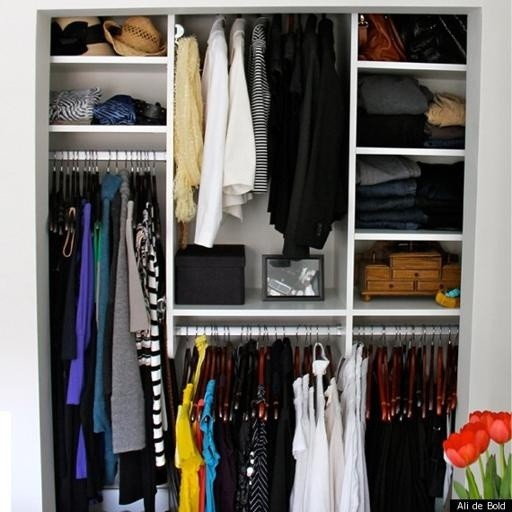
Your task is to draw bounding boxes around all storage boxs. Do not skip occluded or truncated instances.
[174,244,245,307]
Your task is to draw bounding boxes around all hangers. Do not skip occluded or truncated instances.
[174,323,461,422]
[48,149,162,235]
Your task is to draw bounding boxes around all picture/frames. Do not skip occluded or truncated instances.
[261,255,324,302]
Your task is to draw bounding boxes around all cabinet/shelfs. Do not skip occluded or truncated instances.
[37,8,482,511]
[361,253,460,300]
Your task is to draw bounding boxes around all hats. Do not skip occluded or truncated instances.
[104,16,167,56]
[50,16,114,54]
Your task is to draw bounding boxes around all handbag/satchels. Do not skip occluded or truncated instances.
[49,87,102,125]
[358,14,466,64]
[94,95,138,125]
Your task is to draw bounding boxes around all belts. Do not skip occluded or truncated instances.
[139,102,166,125]
